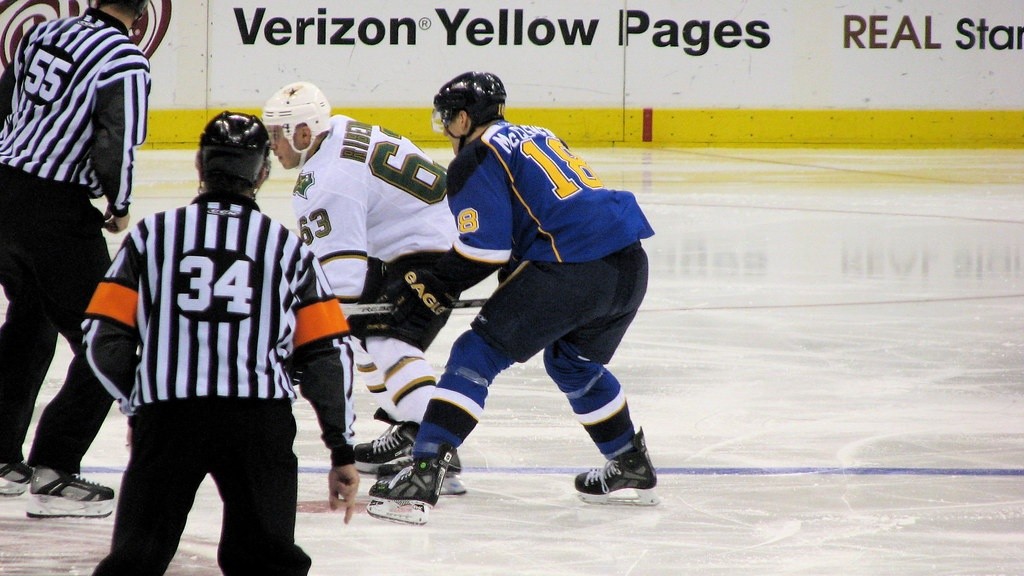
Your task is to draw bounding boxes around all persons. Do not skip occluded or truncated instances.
[81,110,359,576]
[370,72,657,510]
[0,0,151,502]
[261,82,461,479]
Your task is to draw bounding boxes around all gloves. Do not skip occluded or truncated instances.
[381,270,460,338]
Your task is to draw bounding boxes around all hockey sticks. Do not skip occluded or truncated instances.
[339,297,488,319]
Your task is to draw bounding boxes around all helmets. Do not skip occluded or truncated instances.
[199,111,271,184]
[262,82,331,136]
[121,1,149,16]
[434,72,508,124]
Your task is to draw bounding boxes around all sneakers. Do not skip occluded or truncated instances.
[352,421,422,474]
[574,429,656,506]
[377,449,466,495]
[367,441,458,524]
[0,461,35,494]
[27,464,114,518]
